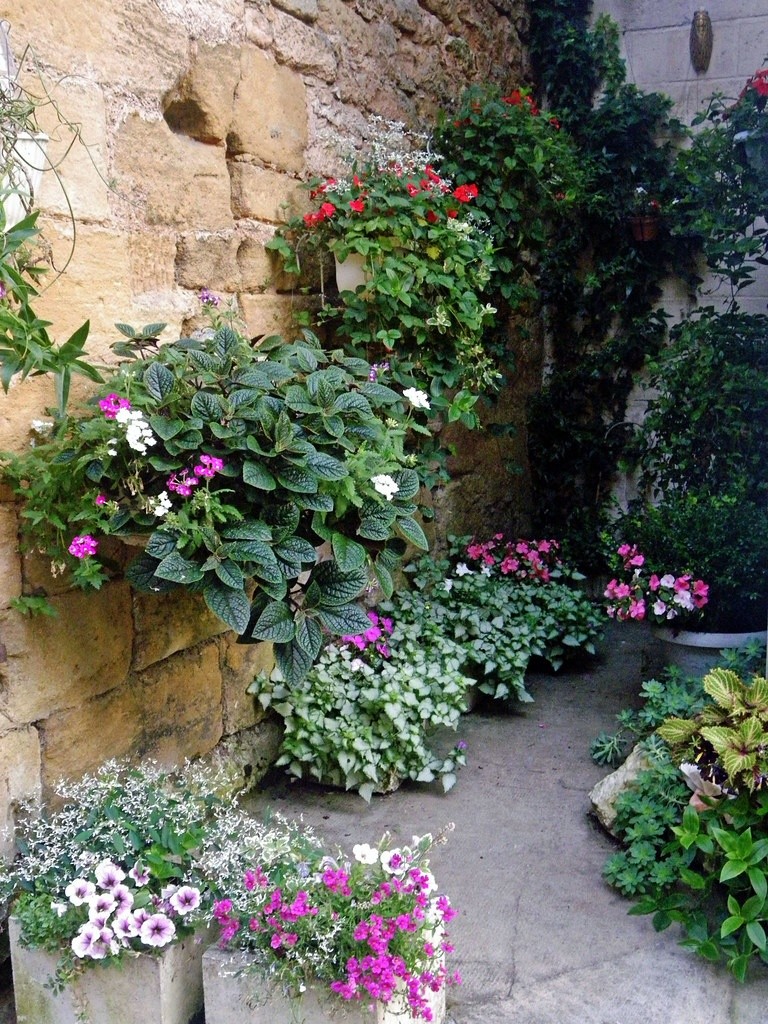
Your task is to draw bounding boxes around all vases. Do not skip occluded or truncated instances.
[330,252,374,301]
[648,629,768,688]
[631,219,660,241]
[0,132,54,234]
[735,138,768,170]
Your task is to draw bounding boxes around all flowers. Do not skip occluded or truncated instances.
[0,0,768,1024]
